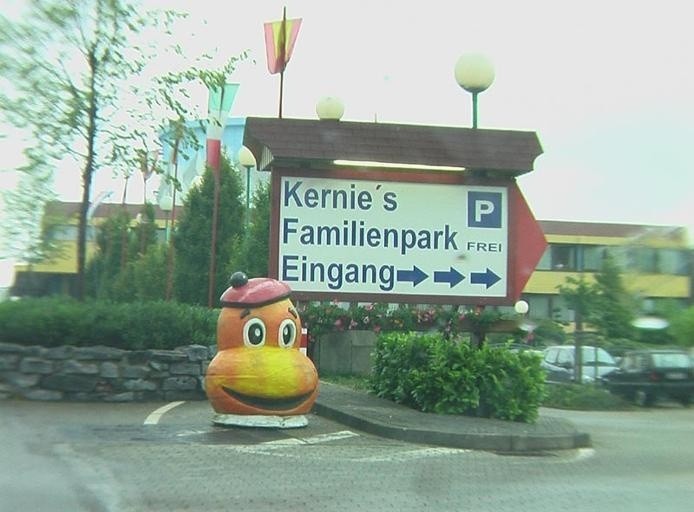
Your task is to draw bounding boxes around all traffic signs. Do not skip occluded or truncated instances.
[267,166,548,306]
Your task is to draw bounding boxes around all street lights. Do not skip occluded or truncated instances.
[238,146,257,230]
[515,300,529,320]
[159,195,173,244]
[455,51,495,129]
[316,95,345,121]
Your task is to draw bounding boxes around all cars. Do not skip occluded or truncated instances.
[509,344,694,407]
